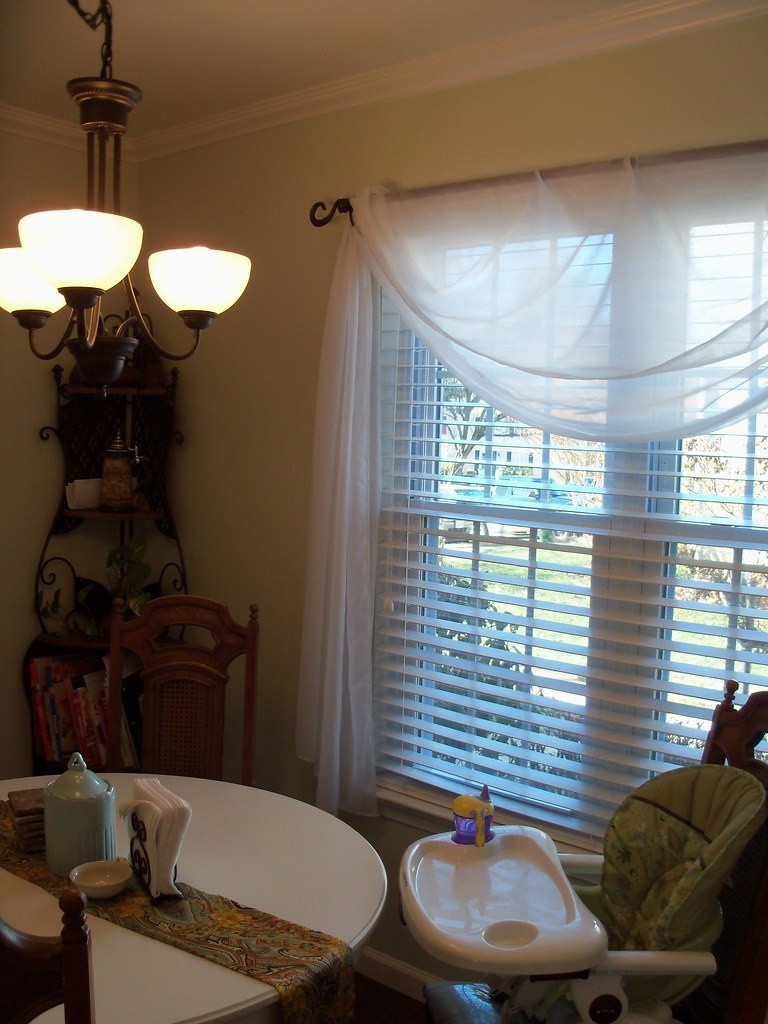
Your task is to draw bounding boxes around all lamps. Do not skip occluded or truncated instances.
[0,0,251,362]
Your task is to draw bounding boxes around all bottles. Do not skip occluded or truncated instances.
[453,784,494,847]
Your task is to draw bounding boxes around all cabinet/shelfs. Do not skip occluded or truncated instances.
[21,308,188,774]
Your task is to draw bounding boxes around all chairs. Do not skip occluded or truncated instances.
[0,885,94,1024]
[421,679,768,1024]
[479,763,768,1024]
[104,589,263,787]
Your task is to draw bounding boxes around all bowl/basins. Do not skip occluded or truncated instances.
[69,860,133,898]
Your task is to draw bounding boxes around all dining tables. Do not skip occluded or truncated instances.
[0,773,388,1023]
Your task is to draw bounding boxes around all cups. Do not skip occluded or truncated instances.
[43,753,117,876]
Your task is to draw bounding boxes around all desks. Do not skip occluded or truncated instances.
[399,818,609,982]
